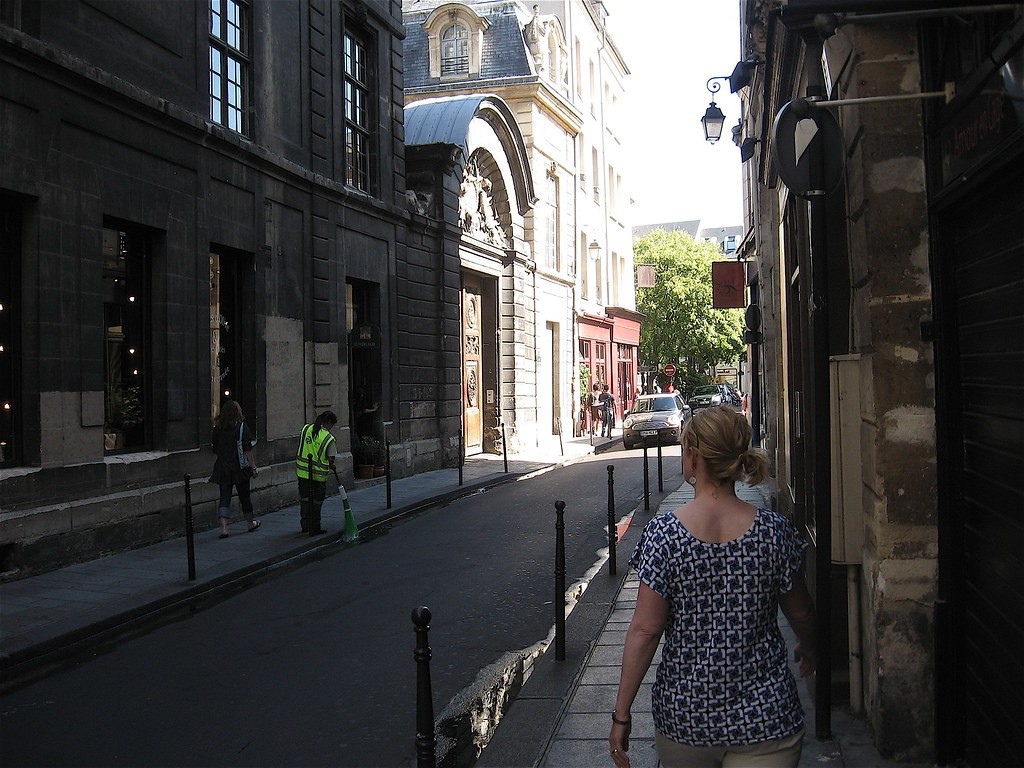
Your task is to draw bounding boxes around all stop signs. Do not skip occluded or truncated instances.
[664,364,676,377]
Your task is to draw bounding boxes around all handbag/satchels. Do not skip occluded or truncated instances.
[237,422,251,467]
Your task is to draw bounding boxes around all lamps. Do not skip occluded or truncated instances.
[701,60,765,164]
[588,238,601,263]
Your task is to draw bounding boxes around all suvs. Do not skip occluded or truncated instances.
[688,385,723,414]
[623,390,692,447]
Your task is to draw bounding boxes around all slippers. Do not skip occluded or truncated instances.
[247,519,261,532]
[219,533,230,539]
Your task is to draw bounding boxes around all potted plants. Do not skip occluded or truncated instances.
[352,436,388,479]
[105,386,143,450]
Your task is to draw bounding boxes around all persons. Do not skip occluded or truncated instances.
[591,384,617,438]
[653,386,658,401]
[209,399,263,539]
[296,410,337,536]
[606,405,820,768]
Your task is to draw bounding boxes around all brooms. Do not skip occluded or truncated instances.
[332,469,360,543]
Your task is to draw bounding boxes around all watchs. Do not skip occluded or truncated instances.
[611,710,632,725]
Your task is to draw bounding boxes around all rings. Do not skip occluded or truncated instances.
[615,749,618,752]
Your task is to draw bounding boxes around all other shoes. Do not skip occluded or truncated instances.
[302,528,327,536]
[592,429,613,438]
